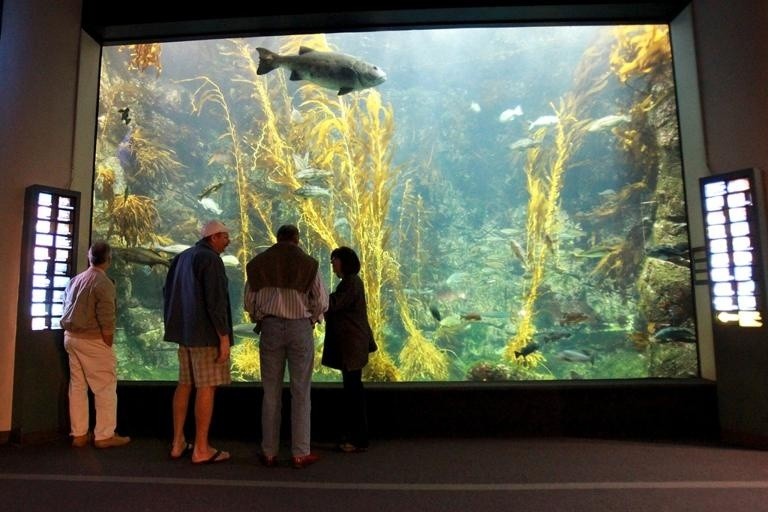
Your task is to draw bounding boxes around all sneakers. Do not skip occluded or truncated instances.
[338,442,369,454]
[71,433,88,449]
[94,433,131,450]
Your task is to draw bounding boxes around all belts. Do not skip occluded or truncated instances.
[262,315,279,320]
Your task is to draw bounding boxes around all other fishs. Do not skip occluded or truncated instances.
[465,223,694,365]
[468,99,482,112]
[496,102,632,151]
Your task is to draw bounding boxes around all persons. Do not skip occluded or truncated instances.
[244,225,330,469]
[60,243,131,448]
[163,219,234,463]
[322,247,378,452]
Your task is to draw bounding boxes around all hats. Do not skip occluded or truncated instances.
[200,218,232,239]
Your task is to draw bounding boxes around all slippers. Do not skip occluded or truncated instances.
[170,439,194,462]
[191,449,232,466]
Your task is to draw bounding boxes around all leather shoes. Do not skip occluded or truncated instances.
[290,453,322,469]
[262,455,278,467]
